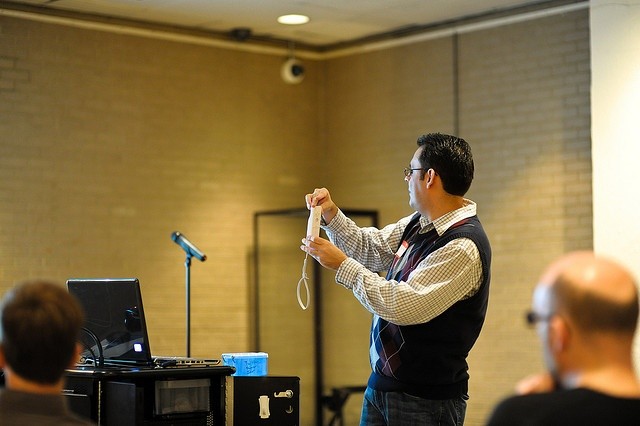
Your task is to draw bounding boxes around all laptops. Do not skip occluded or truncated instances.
[65,280,220,366]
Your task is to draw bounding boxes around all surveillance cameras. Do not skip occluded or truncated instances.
[281,58,312,84]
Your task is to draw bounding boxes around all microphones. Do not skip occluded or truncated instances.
[171,230,208,260]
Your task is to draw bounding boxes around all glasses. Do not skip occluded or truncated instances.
[404,167,438,177]
[527,312,572,331]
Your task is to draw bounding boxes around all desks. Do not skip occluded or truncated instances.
[63,366,299,426]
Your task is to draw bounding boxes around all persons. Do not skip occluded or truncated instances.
[300,132,492,425]
[0,280,96,426]
[484,250,640,426]
